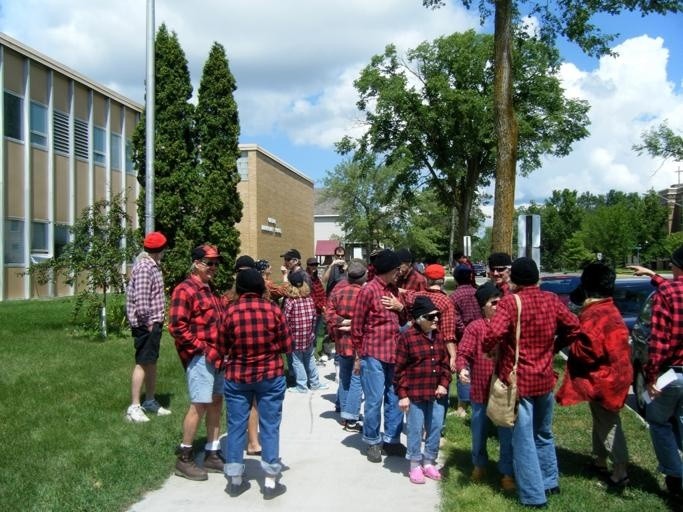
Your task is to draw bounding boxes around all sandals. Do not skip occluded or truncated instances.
[606,478,630,492]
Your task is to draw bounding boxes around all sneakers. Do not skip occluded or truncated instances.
[289,387,306,392]
[143,401,172,415]
[471,465,485,482]
[344,422,441,483]
[321,384,329,389]
[501,476,515,492]
[127,405,150,422]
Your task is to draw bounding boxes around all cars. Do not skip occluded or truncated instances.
[472,264,486,277]
[562,275,678,326]
[535,271,583,306]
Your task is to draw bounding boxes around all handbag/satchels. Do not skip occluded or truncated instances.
[486,379,518,428]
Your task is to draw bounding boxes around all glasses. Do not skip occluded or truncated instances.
[201,261,219,266]
[423,312,440,320]
[337,254,344,257]
[284,258,296,261]
[490,267,507,272]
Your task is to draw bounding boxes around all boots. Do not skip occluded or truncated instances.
[175,446,286,499]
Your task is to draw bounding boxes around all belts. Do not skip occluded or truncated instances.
[662,367,682,372]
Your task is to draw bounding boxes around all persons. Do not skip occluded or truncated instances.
[216,269,295,500]
[126,231,172,422]
[450,251,582,507]
[325,247,456,483]
[627,245,683,495]
[169,245,227,481]
[256,249,329,393]
[220,256,269,455]
[555,260,634,487]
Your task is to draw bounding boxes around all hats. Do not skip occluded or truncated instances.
[236,256,269,294]
[192,245,220,260]
[425,265,446,279]
[144,232,167,252]
[412,296,437,318]
[280,249,318,287]
[671,247,683,268]
[454,252,538,306]
[347,249,411,283]
